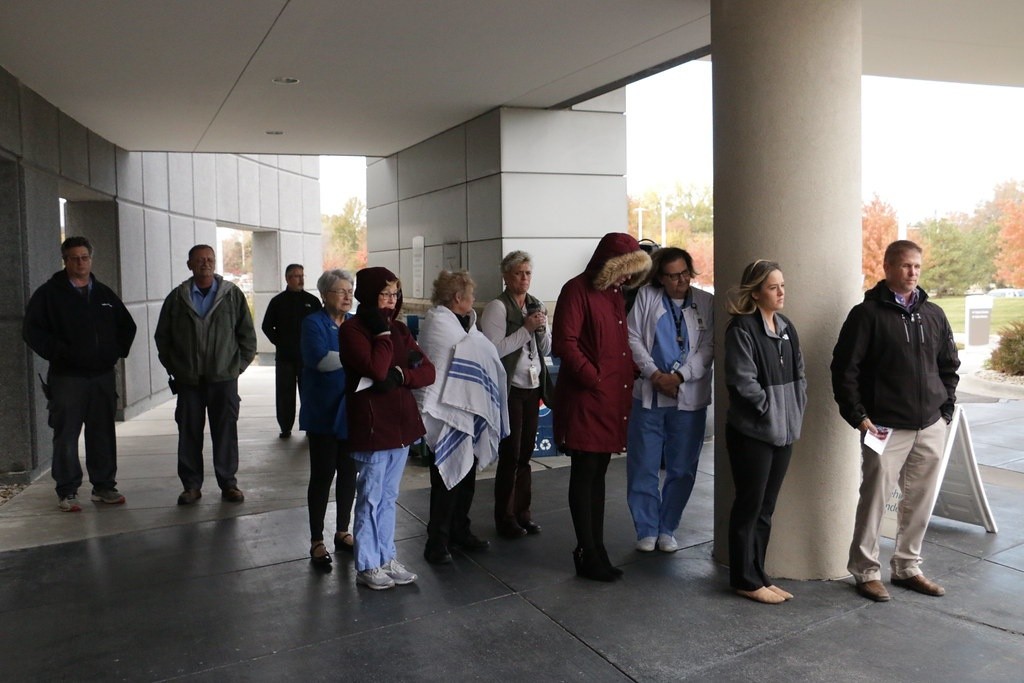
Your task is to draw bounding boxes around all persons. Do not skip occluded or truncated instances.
[626,247,713,552]
[830,240,961,602]
[480,249,554,538]
[155,245,257,504]
[23,236,137,511]
[339,266,436,591]
[724,259,807,603]
[262,264,322,438]
[413,269,510,567]
[300,269,356,566]
[551,233,654,580]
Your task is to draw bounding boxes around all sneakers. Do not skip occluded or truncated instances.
[381,559,417,584]
[91,487,125,502]
[58,493,82,512]
[356,567,395,590]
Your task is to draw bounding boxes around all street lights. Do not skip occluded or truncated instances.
[635,208,645,244]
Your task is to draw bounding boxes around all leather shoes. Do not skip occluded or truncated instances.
[424,533,490,562]
[891,575,945,596]
[334,532,355,547]
[495,521,541,536]
[310,543,332,564]
[856,580,891,600]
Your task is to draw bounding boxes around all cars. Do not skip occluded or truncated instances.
[236,275,253,292]
[988,288,1024,298]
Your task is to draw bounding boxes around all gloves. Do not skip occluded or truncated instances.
[366,307,390,334]
[372,367,403,392]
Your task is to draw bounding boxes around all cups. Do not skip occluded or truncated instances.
[528,302,543,332]
[407,350,424,368]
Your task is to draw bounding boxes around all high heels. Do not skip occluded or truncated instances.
[597,544,623,576]
[574,545,615,581]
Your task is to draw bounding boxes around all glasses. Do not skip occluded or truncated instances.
[661,268,690,280]
[65,255,91,261]
[380,289,400,299]
[326,288,353,296]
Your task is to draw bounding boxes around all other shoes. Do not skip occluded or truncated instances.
[222,487,244,498]
[659,533,678,552]
[178,488,202,503]
[737,585,793,602]
[279,429,291,438]
[636,536,657,551]
